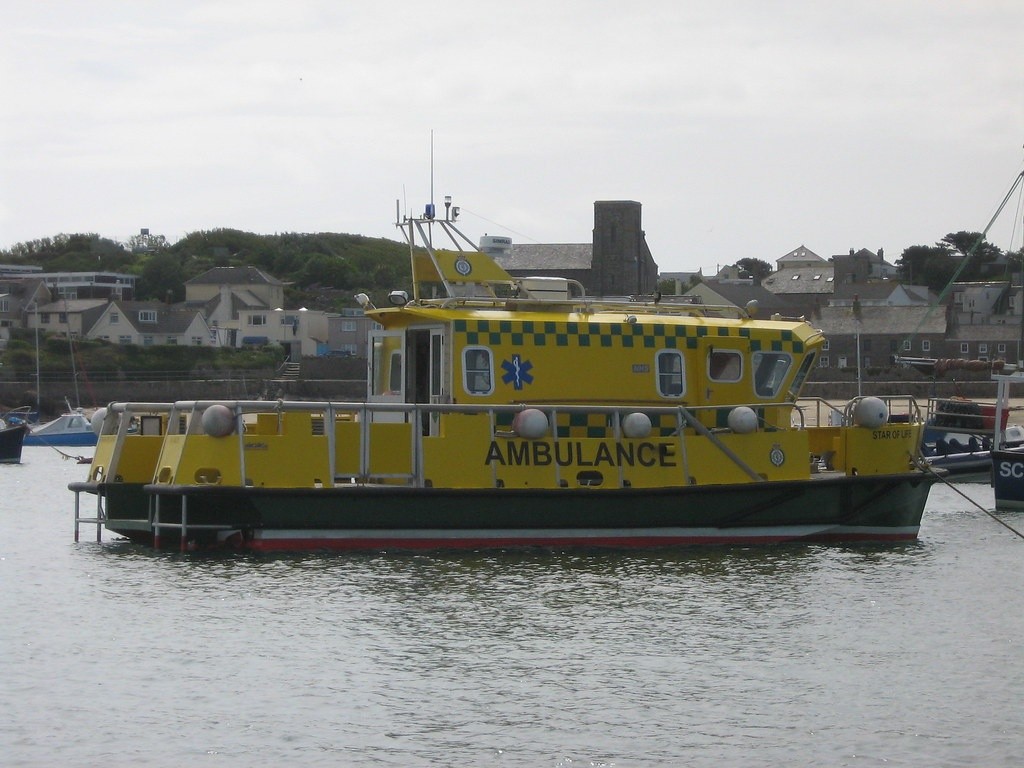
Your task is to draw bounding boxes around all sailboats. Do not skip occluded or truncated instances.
[892,170,1023,379]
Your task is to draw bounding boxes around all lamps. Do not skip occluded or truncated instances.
[354,293,377,309]
[389,291,409,308]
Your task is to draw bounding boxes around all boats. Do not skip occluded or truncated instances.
[989,367,1024,515]
[64,203,954,552]
[20,397,143,447]
[0,405,32,463]
[0,404,40,423]
[815,393,1024,471]
